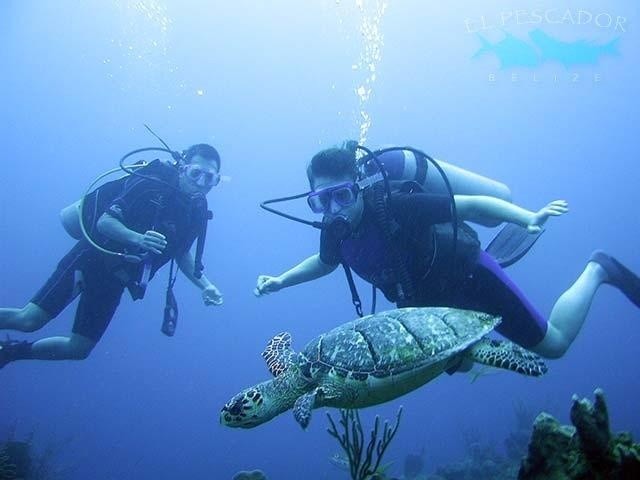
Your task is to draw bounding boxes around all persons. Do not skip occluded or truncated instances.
[0,141,225,372]
[248,145,639,364]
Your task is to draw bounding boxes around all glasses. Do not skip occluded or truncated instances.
[182,164,221,188]
[307,182,360,214]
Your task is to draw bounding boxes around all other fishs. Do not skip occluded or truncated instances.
[328,451,373,475]
